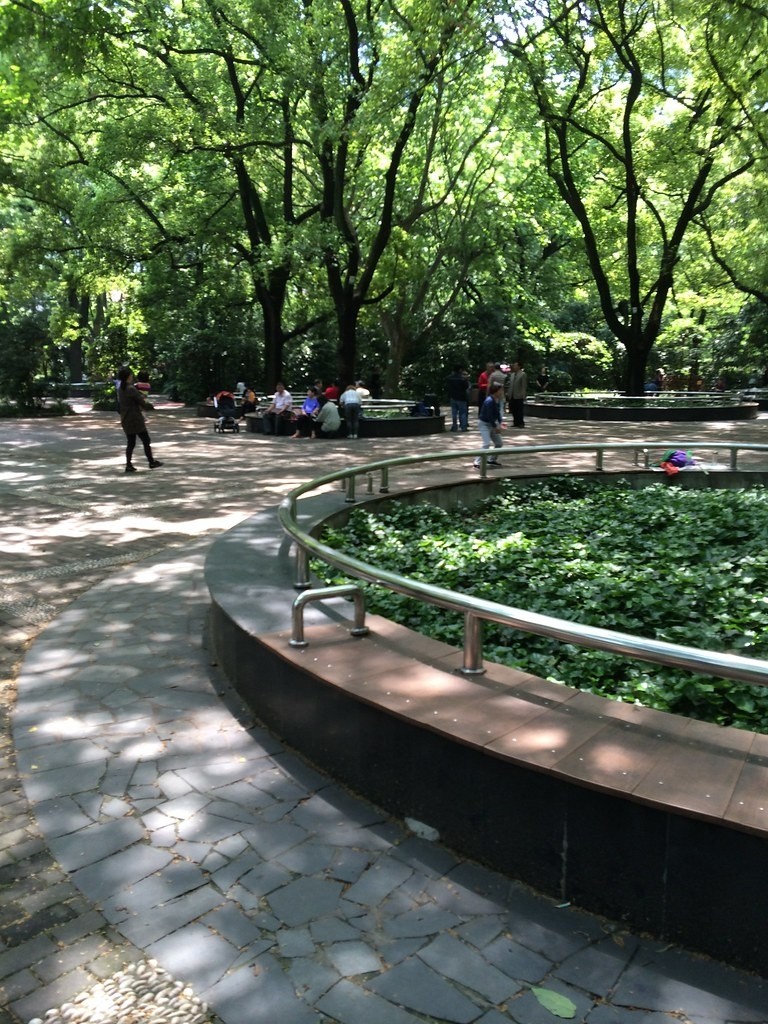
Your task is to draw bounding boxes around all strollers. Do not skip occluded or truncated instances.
[213,390,240,433]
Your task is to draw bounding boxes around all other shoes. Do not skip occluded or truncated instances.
[347,434,358,439]
[149,461,164,468]
[473,465,480,471]
[126,467,137,472]
[487,461,502,466]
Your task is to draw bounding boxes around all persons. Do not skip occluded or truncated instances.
[238,388,256,422]
[447,366,471,432]
[473,381,507,469]
[263,382,292,437]
[536,368,549,393]
[714,378,726,393]
[645,371,663,397]
[479,362,527,428]
[117,367,164,472]
[290,378,371,438]
